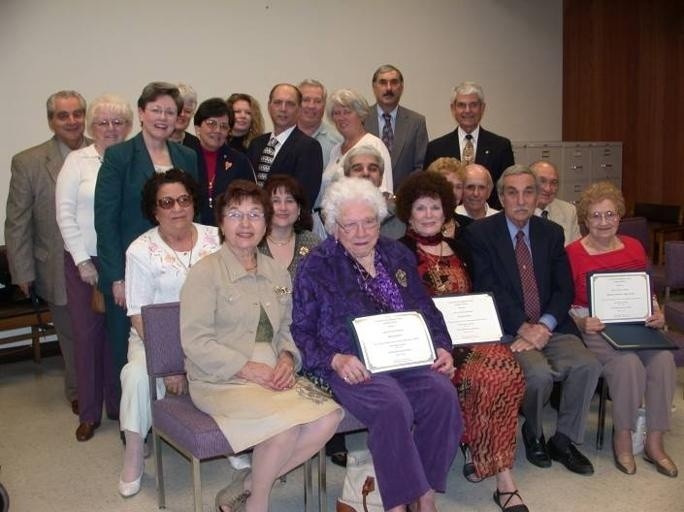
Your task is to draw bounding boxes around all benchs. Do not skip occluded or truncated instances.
[1,245,52,363]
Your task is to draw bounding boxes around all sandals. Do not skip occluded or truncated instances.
[493,488,529,512]
[461,443,483,483]
[215,468,251,512]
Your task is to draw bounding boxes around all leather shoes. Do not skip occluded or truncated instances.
[547,437,594,474]
[611,436,637,474]
[118,463,145,496]
[76,421,100,441]
[72,400,78,414]
[521,421,552,467]
[643,447,677,477]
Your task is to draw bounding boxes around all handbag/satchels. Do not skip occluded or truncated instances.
[336,449,384,512]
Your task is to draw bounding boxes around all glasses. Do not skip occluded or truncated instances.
[159,194,191,209]
[98,119,123,127]
[335,216,378,232]
[227,209,263,220]
[207,119,229,131]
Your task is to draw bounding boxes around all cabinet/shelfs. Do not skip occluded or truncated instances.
[507,140,624,224]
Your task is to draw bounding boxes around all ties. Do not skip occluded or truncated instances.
[541,211,548,218]
[515,231,541,323]
[256,139,278,186]
[381,113,393,154]
[462,135,474,164]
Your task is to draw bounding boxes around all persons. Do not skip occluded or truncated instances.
[527,161,582,247]
[565,182,678,478]
[179,178,344,510]
[392,171,527,509]
[118,166,220,497]
[5,65,516,454]
[464,162,603,474]
[292,177,464,510]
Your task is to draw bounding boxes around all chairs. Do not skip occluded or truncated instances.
[575,218,683,449]
[318,404,368,512]
[139,300,313,512]
